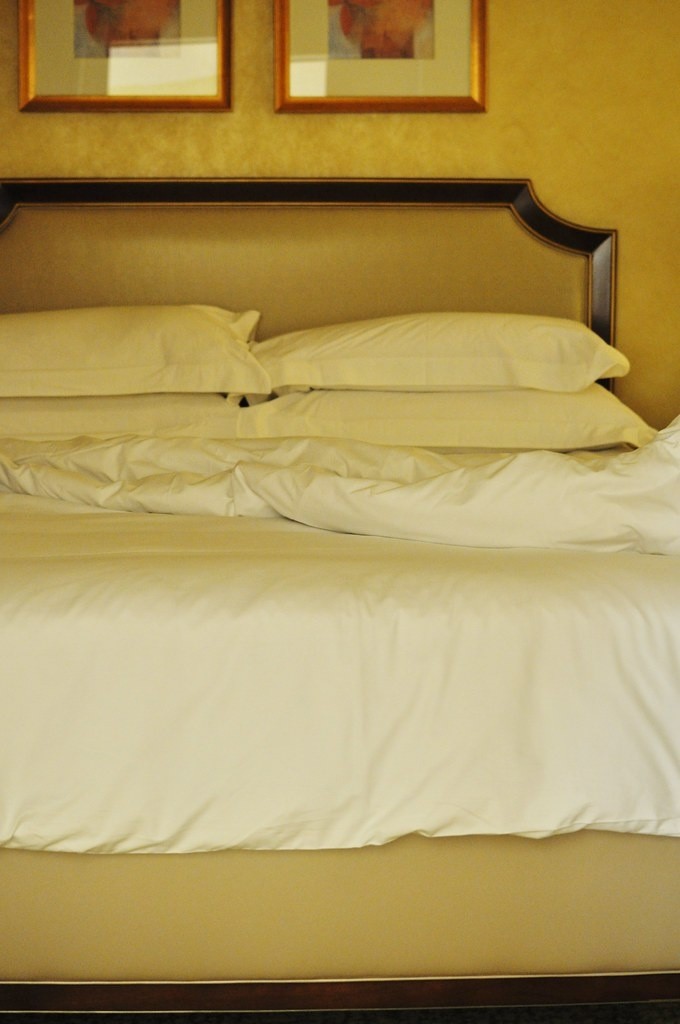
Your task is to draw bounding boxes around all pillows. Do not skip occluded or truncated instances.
[228,313,630,408]
[239,383,658,454]
[0,305,272,395]
[0,393,241,439]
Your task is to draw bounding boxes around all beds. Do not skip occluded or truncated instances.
[0,176,680,1002]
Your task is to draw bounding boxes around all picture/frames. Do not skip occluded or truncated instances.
[273,0,488,115]
[17,0,233,113]
